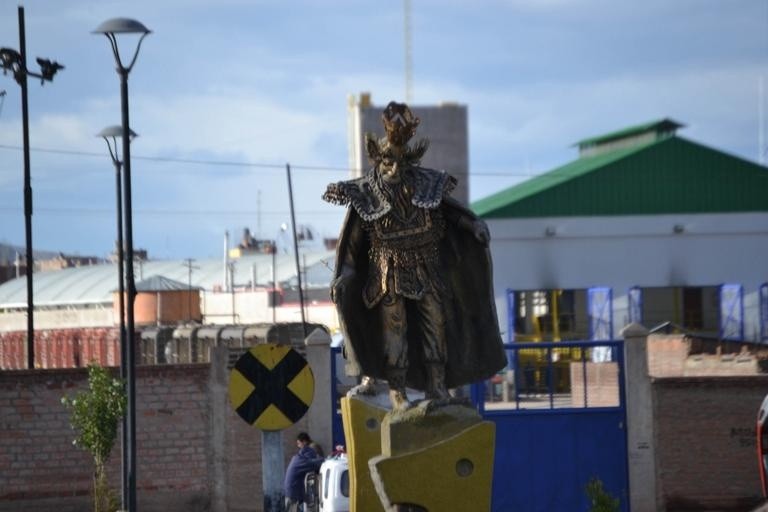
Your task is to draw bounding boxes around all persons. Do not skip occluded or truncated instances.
[320,136,492,413]
[296,431,324,459]
[281,442,326,512]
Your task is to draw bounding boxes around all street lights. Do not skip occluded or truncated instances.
[92,123,136,511]
[225,247,246,322]
[0,5,67,371]
[92,13,159,510]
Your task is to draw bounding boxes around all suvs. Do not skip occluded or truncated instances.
[297,446,353,512]
